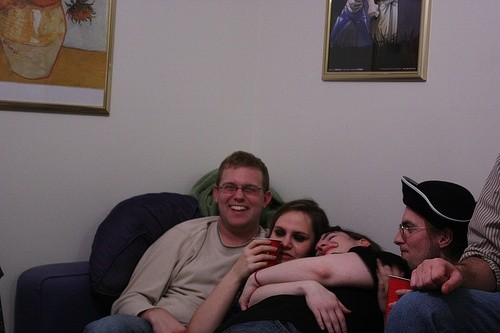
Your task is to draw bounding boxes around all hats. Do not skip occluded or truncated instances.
[401,175,476,229]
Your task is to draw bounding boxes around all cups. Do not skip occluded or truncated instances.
[251,237,282,267]
[384,275,414,326]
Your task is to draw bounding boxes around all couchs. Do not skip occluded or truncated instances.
[15,260,115,333]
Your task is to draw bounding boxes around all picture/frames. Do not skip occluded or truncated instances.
[0,0,116,116]
[322,0,431,82]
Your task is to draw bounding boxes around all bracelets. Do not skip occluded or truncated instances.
[254,272,263,288]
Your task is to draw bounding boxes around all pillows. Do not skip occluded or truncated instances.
[89,193,200,295]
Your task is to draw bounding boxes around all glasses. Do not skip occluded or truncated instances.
[399,223,428,234]
[218,183,265,196]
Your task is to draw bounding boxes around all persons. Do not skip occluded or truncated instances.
[84,151,500,333]
